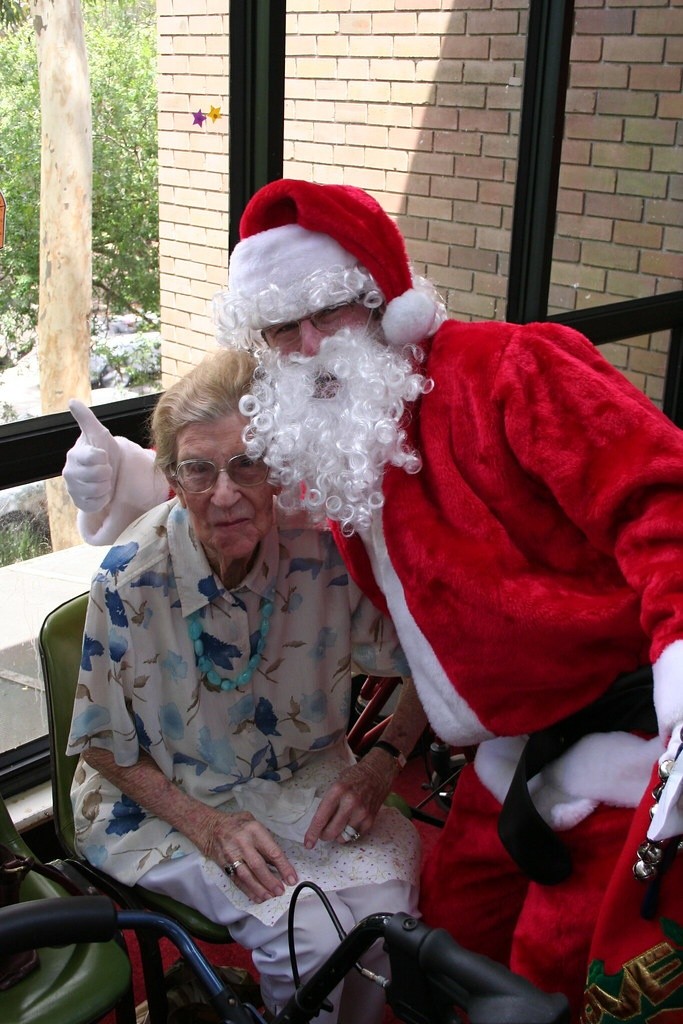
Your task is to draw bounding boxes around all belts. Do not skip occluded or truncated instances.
[498,668,658,887]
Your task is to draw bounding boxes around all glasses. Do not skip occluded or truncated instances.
[169,450,268,495]
[262,293,365,348]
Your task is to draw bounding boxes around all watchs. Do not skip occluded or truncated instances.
[372,740,408,774]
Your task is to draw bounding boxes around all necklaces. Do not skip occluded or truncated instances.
[187,598,273,689]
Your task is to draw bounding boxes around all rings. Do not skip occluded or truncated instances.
[341,825,360,842]
[224,860,242,876]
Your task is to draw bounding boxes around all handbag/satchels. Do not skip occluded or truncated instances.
[0,842,84,992]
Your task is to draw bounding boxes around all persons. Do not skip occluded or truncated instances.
[62,179,683,1024]
[63,350,429,1024]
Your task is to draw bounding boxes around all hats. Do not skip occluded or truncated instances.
[230,178,438,345]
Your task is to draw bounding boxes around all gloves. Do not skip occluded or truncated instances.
[658,718,683,777]
[62,399,122,512]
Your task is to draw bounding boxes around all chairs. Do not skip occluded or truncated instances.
[0,796,138,1024]
[38,591,412,1024]
